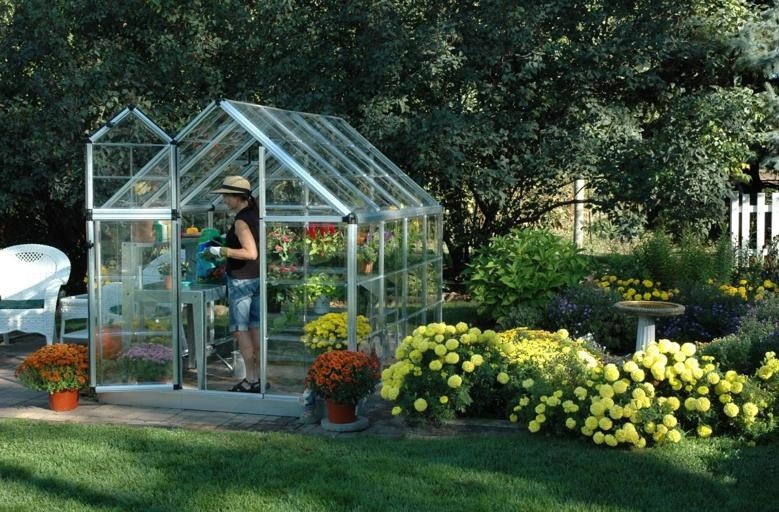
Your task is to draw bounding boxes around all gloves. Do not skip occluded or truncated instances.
[199,245,221,261]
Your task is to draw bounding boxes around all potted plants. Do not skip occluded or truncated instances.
[357,242,379,274]
[303,274,336,315]
[265,271,288,313]
[156,261,178,289]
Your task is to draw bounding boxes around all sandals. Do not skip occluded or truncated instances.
[228,377,272,392]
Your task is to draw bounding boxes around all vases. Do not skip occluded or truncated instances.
[47,386,78,411]
[325,396,358,424]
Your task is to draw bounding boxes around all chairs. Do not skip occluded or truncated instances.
[0,242,125,354]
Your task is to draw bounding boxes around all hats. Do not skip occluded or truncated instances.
[208,175,251,194]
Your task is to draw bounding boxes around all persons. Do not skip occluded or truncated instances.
[203,175,270,393]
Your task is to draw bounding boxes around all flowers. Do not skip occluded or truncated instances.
[307,349,383,404]
[15,336,89,394]
[301,312,373,358]
[115,342,174,381]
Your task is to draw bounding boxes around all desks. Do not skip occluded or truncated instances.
[611,299,687,355]
